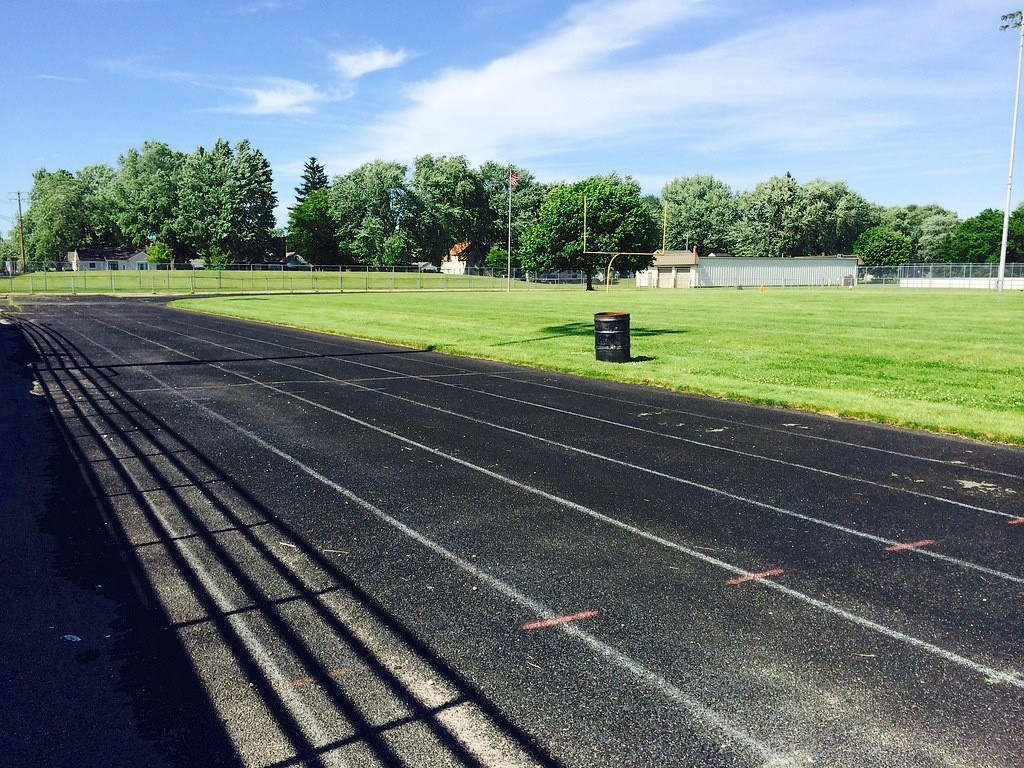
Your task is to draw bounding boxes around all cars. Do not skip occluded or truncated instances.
[417,262,437,272]
[55,262,72,271]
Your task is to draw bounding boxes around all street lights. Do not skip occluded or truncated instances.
[996,11,1023,292]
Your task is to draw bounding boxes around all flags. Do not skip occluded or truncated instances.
[511,172,518,186]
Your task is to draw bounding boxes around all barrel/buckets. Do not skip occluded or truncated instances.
[594,311,631,363]
